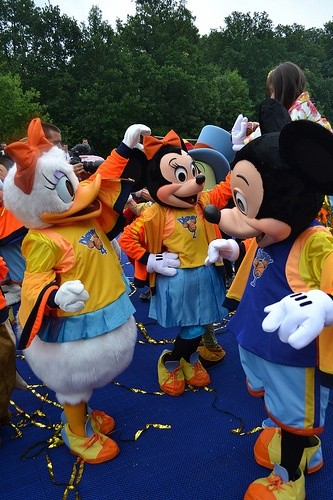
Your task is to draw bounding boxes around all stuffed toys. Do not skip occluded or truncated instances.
[3,118,152,465]
[204,99,333,500]
[0,154,28,430]
[118,113,261,396]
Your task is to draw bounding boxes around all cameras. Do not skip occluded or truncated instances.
[70,155,99,174]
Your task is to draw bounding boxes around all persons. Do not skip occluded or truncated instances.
[0,142,7,150]
[41,122,91,184]
[82,138,96,155]
[267,62,333,133]
